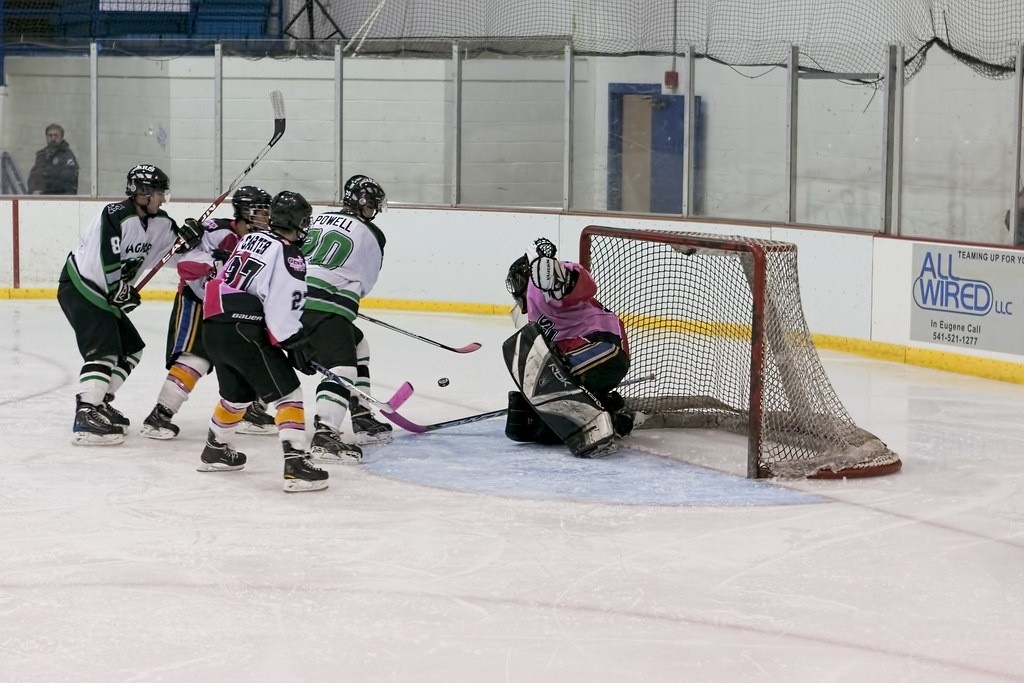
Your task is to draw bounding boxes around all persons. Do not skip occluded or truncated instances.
[27,124,79,195]
[57,164,204,446]
[502,237,646,459]
[140,175,386,492]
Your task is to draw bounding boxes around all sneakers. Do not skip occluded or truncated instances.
[71,393,123,446]
[282,440,329,493]
[349,396,393,444]
[101,393,129,433]
[236,402,280,434]
[308,414,363,466]
[195,427,247,472]
[140,402,180,440]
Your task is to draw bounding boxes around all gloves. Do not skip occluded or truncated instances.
[280,330,322,376]
[530,256,571,301]
[174,218,204,253]
[108,280,141,313]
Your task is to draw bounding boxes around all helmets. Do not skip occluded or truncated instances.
[125,164,170,197]
[343,175,387,220]
[505,253,532,315]
[232,185,272,231]
[269,191,312,238]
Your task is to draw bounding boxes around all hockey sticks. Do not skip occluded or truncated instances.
[357,312,482,354]
[379,372,655,434]
[135,89,287,295]
[311,360,414,414]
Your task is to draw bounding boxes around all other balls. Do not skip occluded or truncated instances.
[438,378,450,388]
